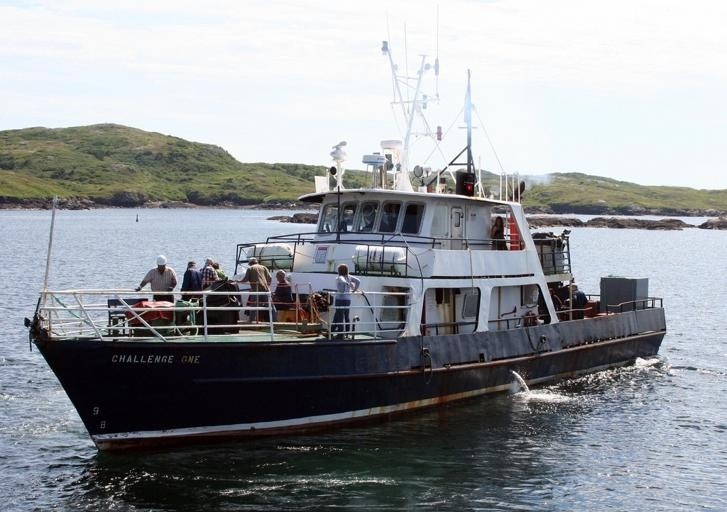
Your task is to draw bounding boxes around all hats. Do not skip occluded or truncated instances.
[205,257,219,269]
[188,261,196,264]
[248,257,258,264]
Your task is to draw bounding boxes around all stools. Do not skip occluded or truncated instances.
[111,315,126,334]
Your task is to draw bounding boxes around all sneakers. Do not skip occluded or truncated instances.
[336,333,350,339]
[242,320,254,323]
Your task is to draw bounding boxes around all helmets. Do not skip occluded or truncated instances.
[156,255,168,265]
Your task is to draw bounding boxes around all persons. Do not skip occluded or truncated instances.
[489,216,508,250]
[571,283,588,319]
[323,264,360,340]
[135,255,295,331]
[549,288,562,311]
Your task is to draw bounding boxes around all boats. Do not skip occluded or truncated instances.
[25,5,667,456]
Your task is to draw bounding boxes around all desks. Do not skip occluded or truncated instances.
[108,298,148,335]
